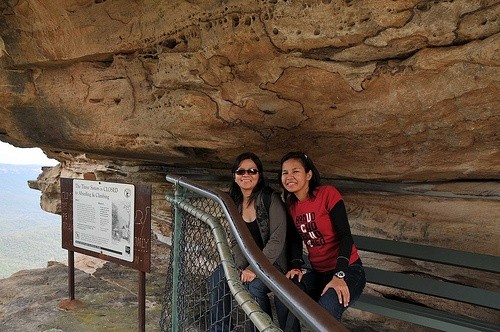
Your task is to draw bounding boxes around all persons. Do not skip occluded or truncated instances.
[274,151,367,332]
[206,151,288,332]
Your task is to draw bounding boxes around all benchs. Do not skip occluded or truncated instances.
[349,232,500,332]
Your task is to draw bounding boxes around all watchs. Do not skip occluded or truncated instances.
[335,270,346,278]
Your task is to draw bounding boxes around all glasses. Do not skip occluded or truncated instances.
[234,168,259,175]
